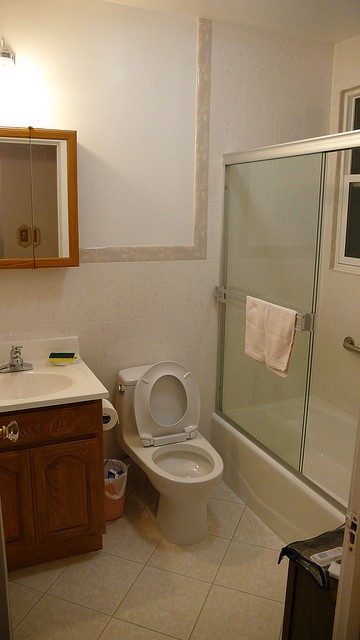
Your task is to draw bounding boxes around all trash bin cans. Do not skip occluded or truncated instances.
[103,459,128,520]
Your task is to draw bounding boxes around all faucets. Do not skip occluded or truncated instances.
[0,345,33,373]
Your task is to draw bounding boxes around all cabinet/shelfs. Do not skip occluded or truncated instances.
[281,557,338,639]
[0,399,107,571]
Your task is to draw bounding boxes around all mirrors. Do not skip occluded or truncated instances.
[1,127,80,268]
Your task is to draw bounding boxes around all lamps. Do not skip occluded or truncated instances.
[0,37,16,65]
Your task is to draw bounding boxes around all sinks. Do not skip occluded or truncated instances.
[0,372,73,401]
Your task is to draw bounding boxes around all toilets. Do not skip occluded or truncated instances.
[113,360,223,545]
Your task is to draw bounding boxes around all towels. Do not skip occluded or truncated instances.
[244,295,298,378]
